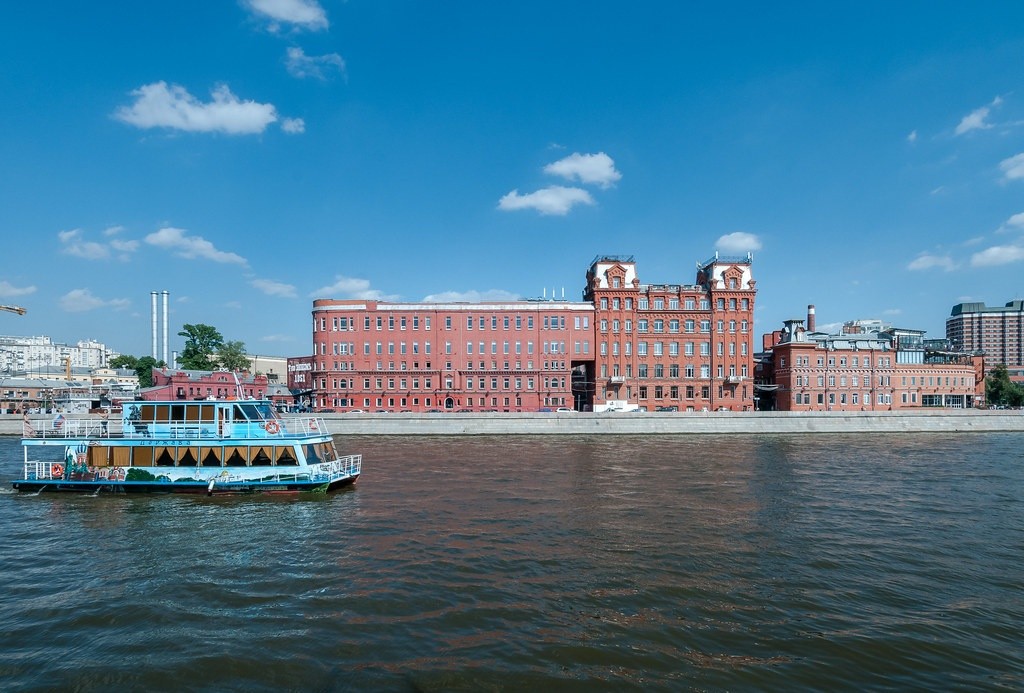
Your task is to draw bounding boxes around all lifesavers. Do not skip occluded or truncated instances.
[310,419,320,430]
[265,422,279,434]
[52,464,63,477]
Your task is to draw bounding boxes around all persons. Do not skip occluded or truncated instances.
[97,409,109,436]
[23,411,36,439]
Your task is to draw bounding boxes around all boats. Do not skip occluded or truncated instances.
[11,372,373,494]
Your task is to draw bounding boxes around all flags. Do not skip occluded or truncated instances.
[53,415,64,430]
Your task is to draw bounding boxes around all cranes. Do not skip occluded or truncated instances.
[0,304,27,316]
